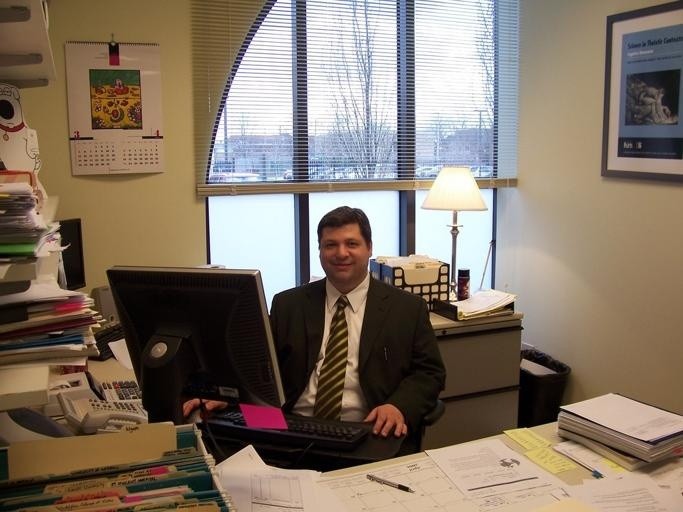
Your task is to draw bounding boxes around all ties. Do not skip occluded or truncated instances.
[313,295,350,421]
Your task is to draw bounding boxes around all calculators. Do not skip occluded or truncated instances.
[98,379,144,400]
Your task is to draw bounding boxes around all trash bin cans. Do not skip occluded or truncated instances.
[517,348,571,429]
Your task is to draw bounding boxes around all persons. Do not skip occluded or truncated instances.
[180,204,446,440]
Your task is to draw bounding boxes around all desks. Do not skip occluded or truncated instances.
[306,421,683,512]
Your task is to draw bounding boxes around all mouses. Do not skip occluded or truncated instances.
[186,402,204,426]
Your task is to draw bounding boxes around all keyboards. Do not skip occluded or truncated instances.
[84,325,125,361]
[201,409,376,450]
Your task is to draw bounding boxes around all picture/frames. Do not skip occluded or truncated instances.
[600,0,683,182]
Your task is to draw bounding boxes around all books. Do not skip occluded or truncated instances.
[0,180,127,376]
[372,253,518,320]
[0,420,235,512]
[553,391,683,473]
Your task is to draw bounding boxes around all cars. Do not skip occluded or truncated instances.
[205,157,494,184]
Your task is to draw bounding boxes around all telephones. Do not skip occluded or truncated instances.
[56,393,149,434]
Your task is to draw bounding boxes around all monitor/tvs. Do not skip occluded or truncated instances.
[53,217,86,290]
[106,265,287,424]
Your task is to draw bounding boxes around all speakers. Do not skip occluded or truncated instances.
[91,285,118,323]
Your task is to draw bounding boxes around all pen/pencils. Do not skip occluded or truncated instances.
[366,475,414,493]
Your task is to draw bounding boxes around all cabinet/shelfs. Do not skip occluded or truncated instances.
[420,318,524,448]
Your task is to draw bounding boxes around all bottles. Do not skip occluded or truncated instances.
[456,267,471,300]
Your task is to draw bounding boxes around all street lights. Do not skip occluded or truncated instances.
[472,109,490,179]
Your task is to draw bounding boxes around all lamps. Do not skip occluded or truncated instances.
[420,167,489,301]
